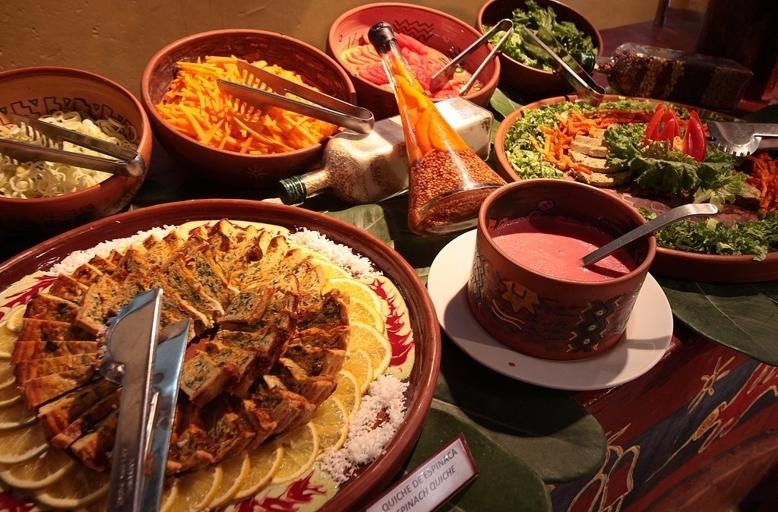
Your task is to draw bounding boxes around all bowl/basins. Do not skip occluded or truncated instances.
[0,67,156,223]
[327,0,501,117]
[0,196,442,510]
[142,27,358,186]
[495,92,776,280]
[468,179,657,361]
[475,1,603,96]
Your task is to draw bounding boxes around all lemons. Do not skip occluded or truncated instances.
[0,216,392,512]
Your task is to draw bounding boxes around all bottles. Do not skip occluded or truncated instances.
[579,43,756,110]
[279,96,495,205]
[366,22,511,249]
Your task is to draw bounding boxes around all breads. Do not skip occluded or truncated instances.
[566,125,633,187]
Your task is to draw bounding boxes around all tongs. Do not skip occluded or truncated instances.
[97,285,191,510]
[426,17,514,97]
[218,60,376,136]
[0,114,145,182]
[708,118,778,162]
[521,16,611,105]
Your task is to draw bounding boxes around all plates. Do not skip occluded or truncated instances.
[426,225,676,392]
